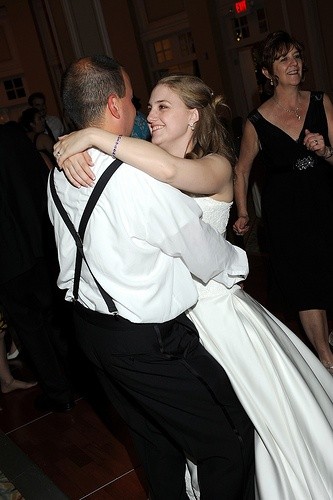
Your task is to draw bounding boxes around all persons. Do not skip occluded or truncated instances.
[0,306,38,394]
[19,109,56,170]
[128,95,149,140]
[53,74,333,500]
[47,56,256,500]
[0,120,97,411]
[232,30,333,377]
[28,92,65,139]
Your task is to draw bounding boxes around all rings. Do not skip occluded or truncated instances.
[314,140,318,145]
[57,152,61,156]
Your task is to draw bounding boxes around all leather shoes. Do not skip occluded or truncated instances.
[33,394,74,411]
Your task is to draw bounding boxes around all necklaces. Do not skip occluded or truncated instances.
[280,102,301,120]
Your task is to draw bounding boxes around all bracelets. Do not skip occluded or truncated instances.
[112,135,122,158]
[326,148,333,159]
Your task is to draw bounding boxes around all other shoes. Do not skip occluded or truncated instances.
[7,349,19,360]
